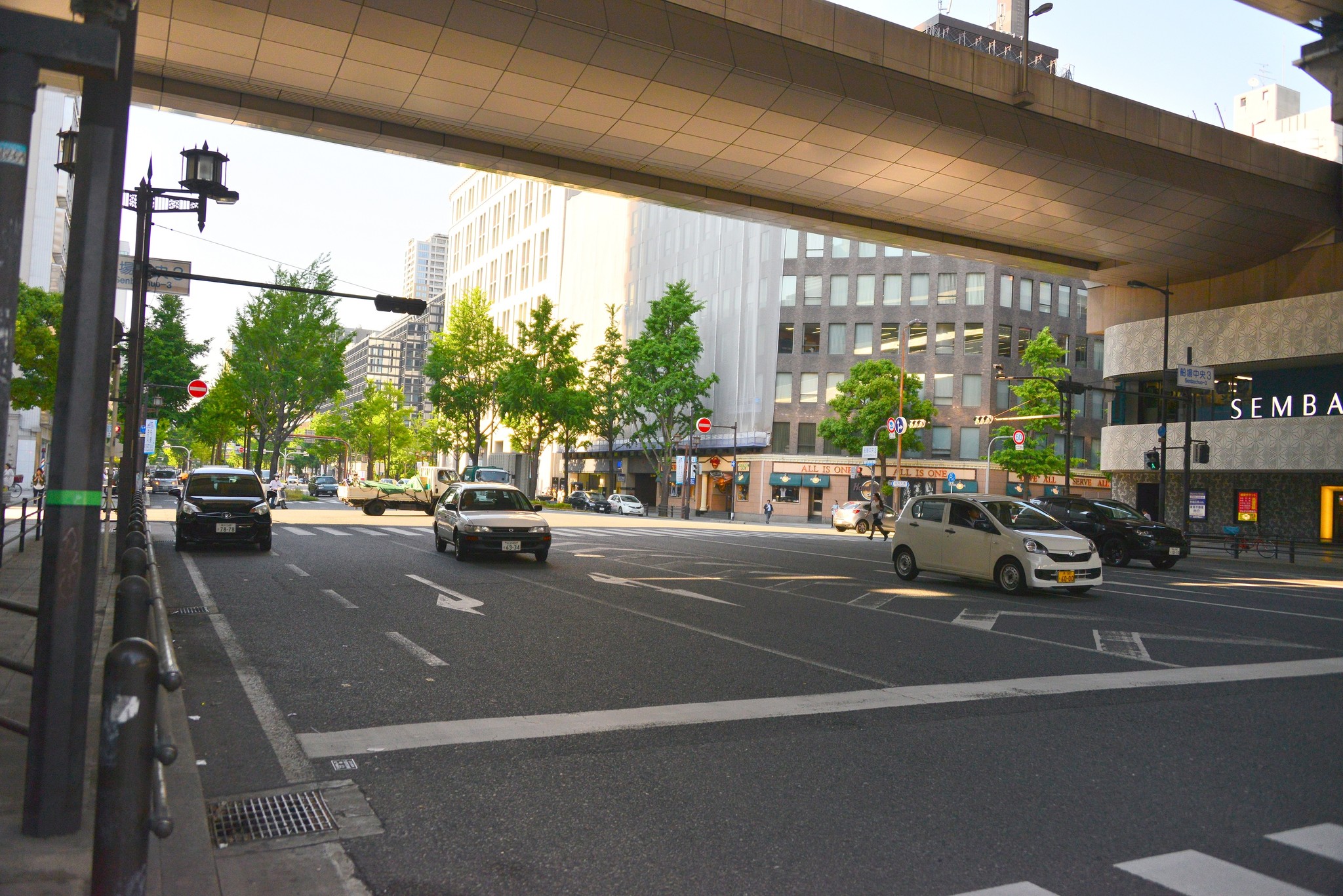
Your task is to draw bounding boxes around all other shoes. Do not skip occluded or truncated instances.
[285,507,288,509]
[766,521,769,524]
[270,507,273,509]
[831,526,835,528]
[883,536,888,541]
[867,536,872,540]
[33,503,36,506]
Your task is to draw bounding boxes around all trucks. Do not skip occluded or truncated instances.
[337,466,463,516]
[460,465,515,485]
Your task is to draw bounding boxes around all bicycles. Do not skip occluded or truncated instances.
[339,467,360,487]
[8,483,22,498]
[1223,521,1276,558]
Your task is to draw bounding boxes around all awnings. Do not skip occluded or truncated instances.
[802,474,831,488]
[1005,482,1032,497]
[654,472,677,482]
[768,471,802,486]
[943,479,978,494]
[734,471,750,484]
[1043,484,1062,496]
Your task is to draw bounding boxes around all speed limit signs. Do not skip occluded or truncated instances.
[1013,429,1025,445]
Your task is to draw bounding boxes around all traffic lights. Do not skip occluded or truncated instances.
[1201,446,1209,462]
[693,464,698,474]
[910,421,923,427]
[114,426,120,432]
[975,416,991,424]
[1149,452,1158,468]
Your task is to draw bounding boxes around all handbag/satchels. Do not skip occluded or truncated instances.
[877,507,884,519]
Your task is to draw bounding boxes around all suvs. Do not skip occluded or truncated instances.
[567,490,612,514]
[1014,494,1189,569]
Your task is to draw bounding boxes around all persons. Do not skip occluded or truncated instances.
[2,463,14,491]
[32,468,46,508]
[864,492,888,541]
[1141,508,1151,521]
[541,485,579,504]
[830,500,840,528]
[103,464,142,490]
[269,474,287,508]
[958,503,982,529]
[763,499,774,524]
[437,471,456,484]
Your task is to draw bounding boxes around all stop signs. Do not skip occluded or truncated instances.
[239,448,244,454]
[188,379,209,399]
[697,418,712,433]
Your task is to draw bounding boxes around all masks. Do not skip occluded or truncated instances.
[275,477,278,480]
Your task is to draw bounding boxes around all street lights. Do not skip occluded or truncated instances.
[140,394,164,426]
[1021,3,1054,94]
[58,123,230,571]
[673,433,701,518]
[893,318,926,514]
[415,450,430,466]
[523,442,544,462]
[473,448,484,458]
[131,188,241,501]
[1127,268,1174,524]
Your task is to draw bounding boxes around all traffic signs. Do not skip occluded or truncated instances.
[1177,363,1215,390]
[116,254,192,297]
[731,461,735,467]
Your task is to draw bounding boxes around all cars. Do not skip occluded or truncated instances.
[258,467,309,486]
[891,493,1103,596]
[167,467,277,551]
[308,476,339,498]
[379,478,397,485]
[833,501,901,534]
[395,478,410,487]
[607,494,644,517]
[432,481,551,562]
[139,461,185,495]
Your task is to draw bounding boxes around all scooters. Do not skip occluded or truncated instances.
[264,481,287,509]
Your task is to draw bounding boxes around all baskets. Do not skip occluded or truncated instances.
[1222,526,1239,534]
[14,474,24,482]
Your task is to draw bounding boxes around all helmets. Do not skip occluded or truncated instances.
[273,474,279,479]
